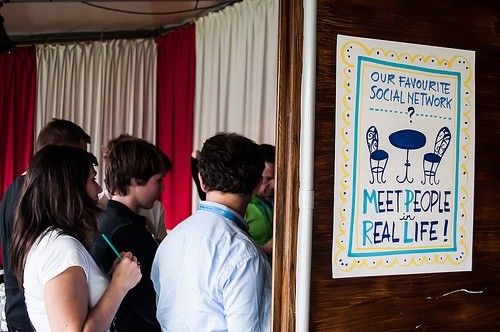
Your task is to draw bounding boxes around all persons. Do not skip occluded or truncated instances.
[0,118,91,332]
[83,134,167,253]
[10,146,142,332]
[190,149,206,201]
[243,144,276,268]
[90,139,172,332]
[150,133,273,332]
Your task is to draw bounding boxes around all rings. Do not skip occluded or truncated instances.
[137,261,141,266]
